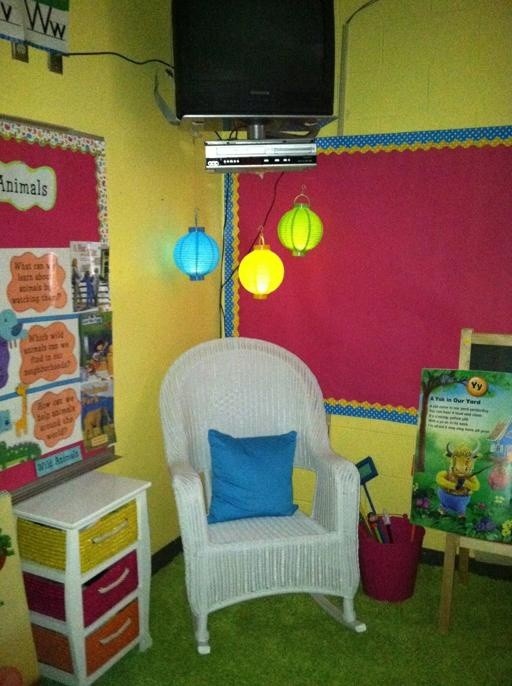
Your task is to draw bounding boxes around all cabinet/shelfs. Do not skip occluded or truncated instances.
[12,469,154,685]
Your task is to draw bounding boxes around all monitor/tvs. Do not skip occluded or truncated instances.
[170,0,334,120]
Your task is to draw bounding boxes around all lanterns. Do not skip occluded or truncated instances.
[238,244,284,300]
[172,227,220,281]
[277,202,324,256]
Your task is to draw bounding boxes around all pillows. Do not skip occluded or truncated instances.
[207,429,297,525]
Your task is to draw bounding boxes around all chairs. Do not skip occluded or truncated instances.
[159,337,369,657]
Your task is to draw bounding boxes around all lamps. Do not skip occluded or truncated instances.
[277,169,324,258]
[173,127,220,281]
[236,171,284,300]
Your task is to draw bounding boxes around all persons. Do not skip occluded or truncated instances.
[72,258,107,308]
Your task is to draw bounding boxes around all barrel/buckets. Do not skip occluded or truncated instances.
[359,516,425,602]
